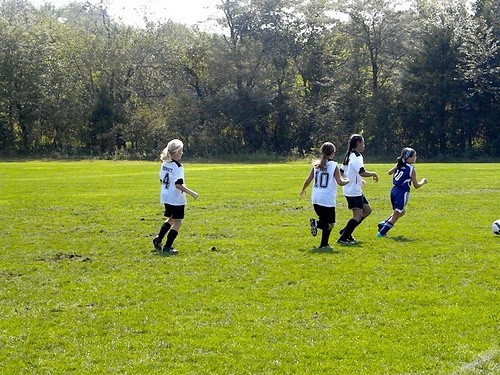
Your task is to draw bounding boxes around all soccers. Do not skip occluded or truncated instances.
[491,221,500,235]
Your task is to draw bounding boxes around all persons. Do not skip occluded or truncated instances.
[377,147,428,238]
[337,134,378,245]
[300,142,350,249]
[153,139,199,255]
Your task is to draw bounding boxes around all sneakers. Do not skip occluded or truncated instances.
[319,244,334,249]
[378,221,385,232]
[339,229,354,241]
[377,232,388,237]
[310,218,318,237]
[153,236,162,253]
[338,236,355,245]
[162,245,178,254]
